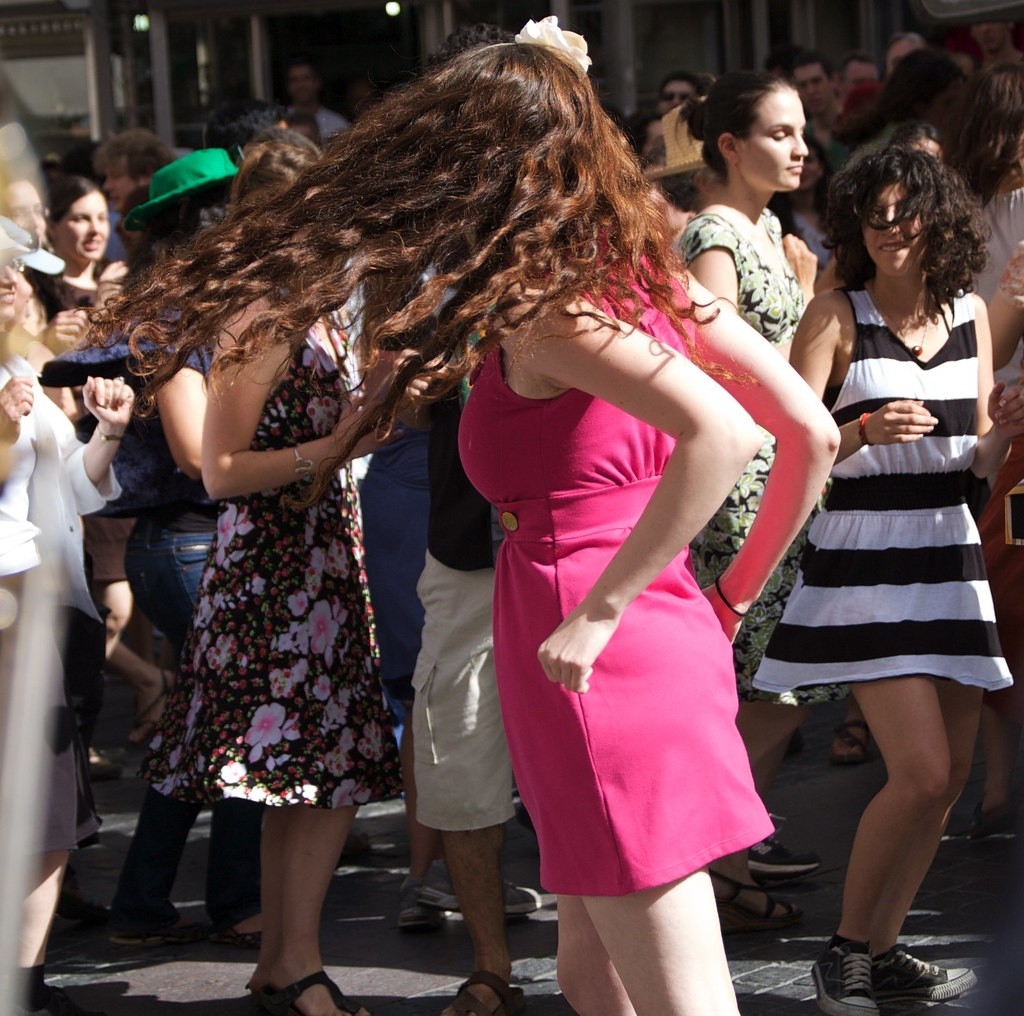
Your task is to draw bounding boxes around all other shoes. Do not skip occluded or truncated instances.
[52,886,112,934]
[338,820,373,856]
[85,744,120,781]
[209,916,263,949]
[74,809,99,844]
[110,913,211,946]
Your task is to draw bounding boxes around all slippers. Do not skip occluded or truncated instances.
[965,795,1018,839]
[245,970,372,1016]
[444,970,525,1016]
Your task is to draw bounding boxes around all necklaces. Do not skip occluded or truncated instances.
[871,281,930,355]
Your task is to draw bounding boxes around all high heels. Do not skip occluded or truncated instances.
[707,865,803,930]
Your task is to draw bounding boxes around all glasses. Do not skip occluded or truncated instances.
[659,92,693,102]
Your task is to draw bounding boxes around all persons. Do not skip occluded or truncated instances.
[0,14,1024,1016]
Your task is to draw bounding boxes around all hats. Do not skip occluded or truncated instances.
[834,79,885,142]
[42,144,84,175]
[0,217,66,275]
[125,148,239,231]
[642,95,711,181]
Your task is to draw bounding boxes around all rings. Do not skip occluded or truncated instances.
[114,376,124,383]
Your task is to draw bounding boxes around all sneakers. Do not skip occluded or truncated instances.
[398,874,444,931]
[5,984,106,1016]
[747,811,820,878]
[812,934,878,1015]
[415,859,538,915]
[870,945,978,1005]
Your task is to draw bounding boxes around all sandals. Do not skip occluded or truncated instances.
[830,717,871,763]
[129,667,174,744]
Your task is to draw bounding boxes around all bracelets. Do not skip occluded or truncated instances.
[292,448,314,478]
[858,412,872,446]
[96,426,123,441]
[713,574,747,618]
